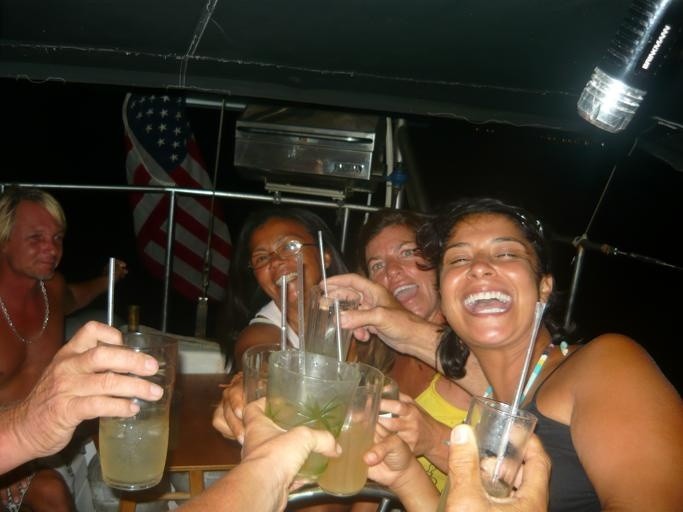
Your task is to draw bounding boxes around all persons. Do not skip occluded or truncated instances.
[165,393,554,512]
[210,205,384,449]
[356,205,491,496]
[1,320,165,477]
[1,181,128,512]
[317,268,492,402]
[356,196,682,512]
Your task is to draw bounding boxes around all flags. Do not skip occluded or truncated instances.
[118,90,234,304]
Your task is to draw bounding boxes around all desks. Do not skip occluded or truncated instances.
[117,373,241,510]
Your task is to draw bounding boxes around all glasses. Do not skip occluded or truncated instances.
[245,240,321,271]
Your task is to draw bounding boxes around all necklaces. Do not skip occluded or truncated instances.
[1,278,50,343]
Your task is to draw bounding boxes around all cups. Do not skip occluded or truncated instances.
[454,393,538,497]
[379,374,398,438]
[306,285,363,362]
[317,360,391,497]
[266,348,362,486]
[97,331,179,492]
[239,341,291,403]
